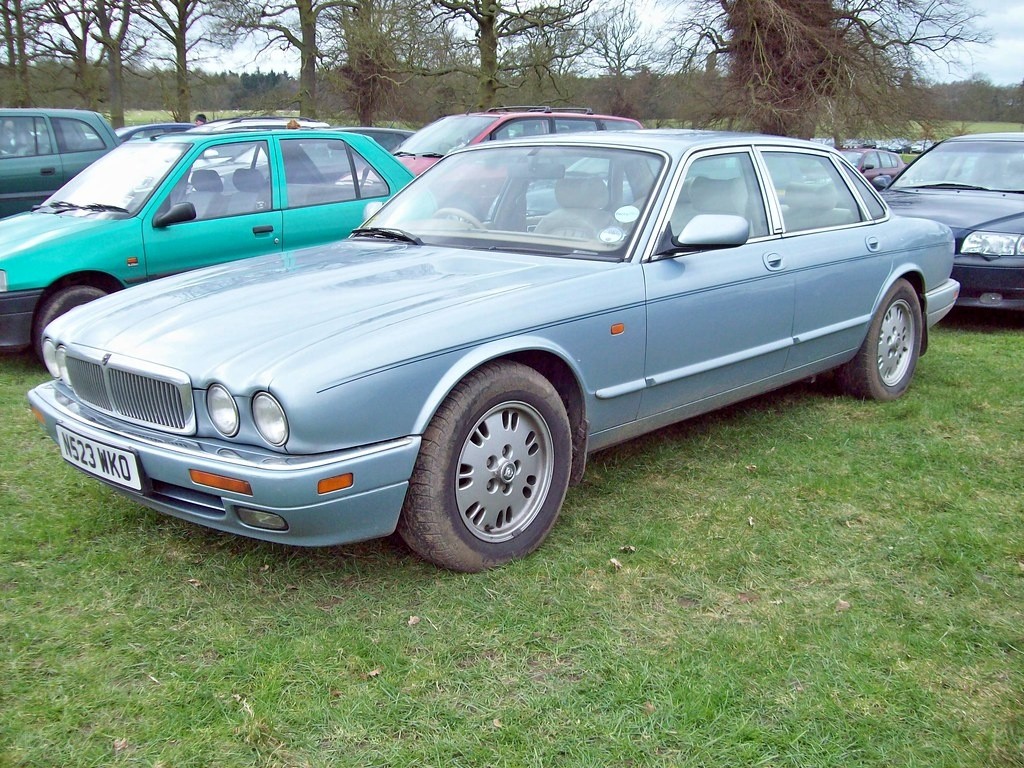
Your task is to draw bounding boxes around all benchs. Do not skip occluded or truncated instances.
[261,185,387,206]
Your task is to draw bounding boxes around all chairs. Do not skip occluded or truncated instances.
[181,170,224,221]
[530,176,613,241]
[659,179,846,237]
[15,131,33,157]
[670,177,751,240]
[217,169,268,218]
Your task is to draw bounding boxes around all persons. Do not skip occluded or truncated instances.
[194,114,207,127]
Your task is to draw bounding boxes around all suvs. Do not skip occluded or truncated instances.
[333,103,646,198]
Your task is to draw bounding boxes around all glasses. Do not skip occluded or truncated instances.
[195,120,201,122]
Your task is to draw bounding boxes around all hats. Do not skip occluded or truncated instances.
[196,114,206,123]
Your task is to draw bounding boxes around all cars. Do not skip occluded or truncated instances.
[784,149,909,207]
[150,116,330,185]
[809,137,932,153]
[486,153,745,223]
[29,128,961,573]
[0,109,126,221]
[884,133,1024,310]
[85,120,203,140]
[193,127,414,193]
[0,124,439,371]
